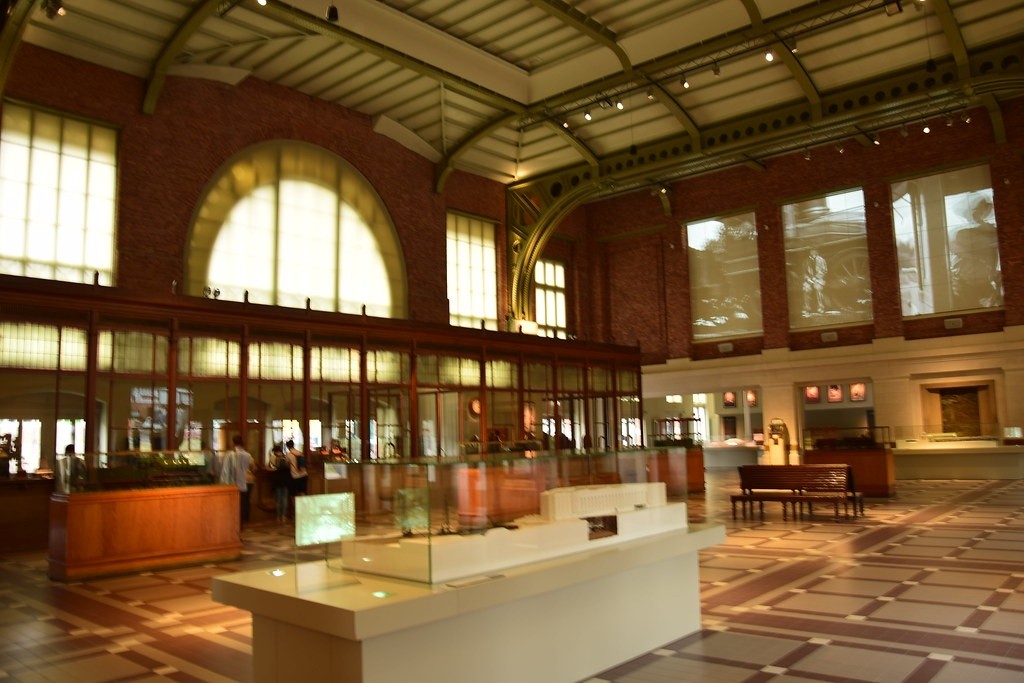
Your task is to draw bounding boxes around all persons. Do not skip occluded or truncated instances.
[54,444,87,495]
[285,439,308,509]
[202,435,256,533]
[269,446,290,523]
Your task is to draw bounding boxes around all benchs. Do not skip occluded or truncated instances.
[730,463,865,523]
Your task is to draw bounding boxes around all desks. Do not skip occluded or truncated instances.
[213,522,727,683]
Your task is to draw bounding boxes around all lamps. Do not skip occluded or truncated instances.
[40,0,67,19]
[324,0,338,22]
